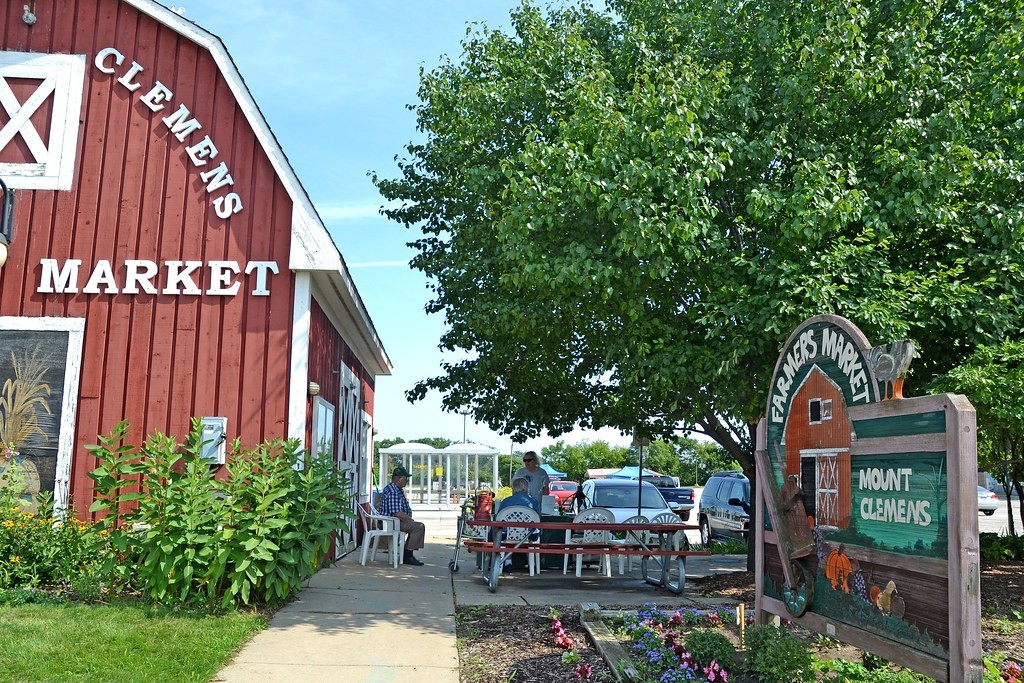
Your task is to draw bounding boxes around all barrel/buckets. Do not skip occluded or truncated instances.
[476,486,493,521]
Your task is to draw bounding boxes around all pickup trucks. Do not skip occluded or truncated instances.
[607,473,695,521]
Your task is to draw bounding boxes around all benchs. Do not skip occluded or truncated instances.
[464,542,712,594]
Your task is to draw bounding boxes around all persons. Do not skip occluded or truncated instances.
[494,478,541,573]
[380,467,425,566]
[511,451,549,569]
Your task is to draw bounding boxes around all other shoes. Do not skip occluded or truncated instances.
[403,555,424,567]
[504,564,512,574]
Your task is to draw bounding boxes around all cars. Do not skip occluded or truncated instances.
[477,482,487,492]
[442,481,447,489]
[569,478,683,546]
[451,480,465,489]
[547,481,579,510]
[977,485,1000,516]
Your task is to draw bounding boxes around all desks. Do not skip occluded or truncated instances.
[477,514,576,571]
[466,520,703,593]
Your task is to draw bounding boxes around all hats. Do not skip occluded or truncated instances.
[392,467,412,477]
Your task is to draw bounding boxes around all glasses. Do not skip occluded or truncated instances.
[523,458,536,462]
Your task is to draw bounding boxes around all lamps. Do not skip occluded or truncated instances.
[0,178,14,270]
[307,380,320,398]
[372,428,378,437]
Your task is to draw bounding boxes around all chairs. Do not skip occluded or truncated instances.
[552,486,557,491]
[608,495,618,504]
[563,507,684,576]
[496,505,541,576]
[356,501,407,568]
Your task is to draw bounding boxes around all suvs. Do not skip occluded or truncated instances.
[697,470,753,547]
